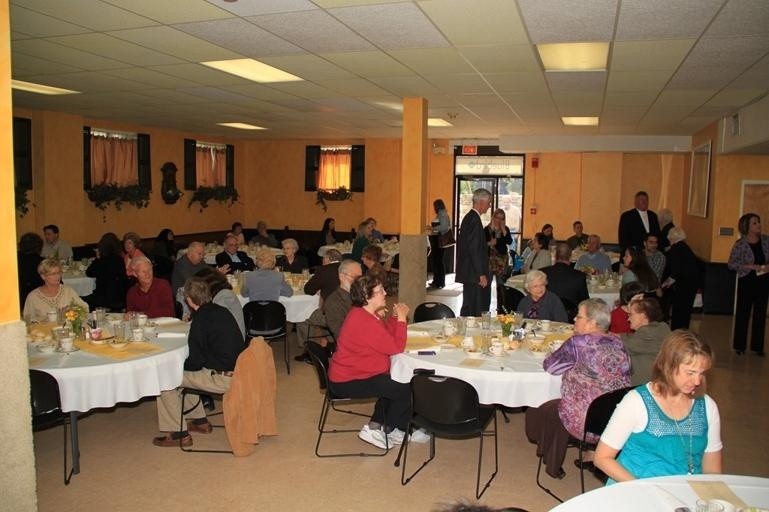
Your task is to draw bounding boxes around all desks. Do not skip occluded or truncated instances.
[548,475,768,512]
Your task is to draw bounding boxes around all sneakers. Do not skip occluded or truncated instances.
[358,425,431,449]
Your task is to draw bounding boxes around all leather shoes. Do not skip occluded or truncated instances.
[187,421,212,433]
[575,460,593,471]
[155,433,193,446]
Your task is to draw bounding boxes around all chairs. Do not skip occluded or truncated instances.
[301,339,390,459]
[271,242,644,422]
[400,372,498,501]
[534,383,653,503]
[24,243,289,485]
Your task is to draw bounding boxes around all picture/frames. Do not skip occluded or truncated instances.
[737,180,769,239]
[686,139,714,220]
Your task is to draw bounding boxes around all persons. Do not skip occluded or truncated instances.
[728,214,769,356]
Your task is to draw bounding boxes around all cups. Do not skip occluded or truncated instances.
[588,268,623,289]
[696,499,769,512]
[59,256,94,275]
[24,307,160,354]
[225,270,314,293]
[432,311,577,356]
